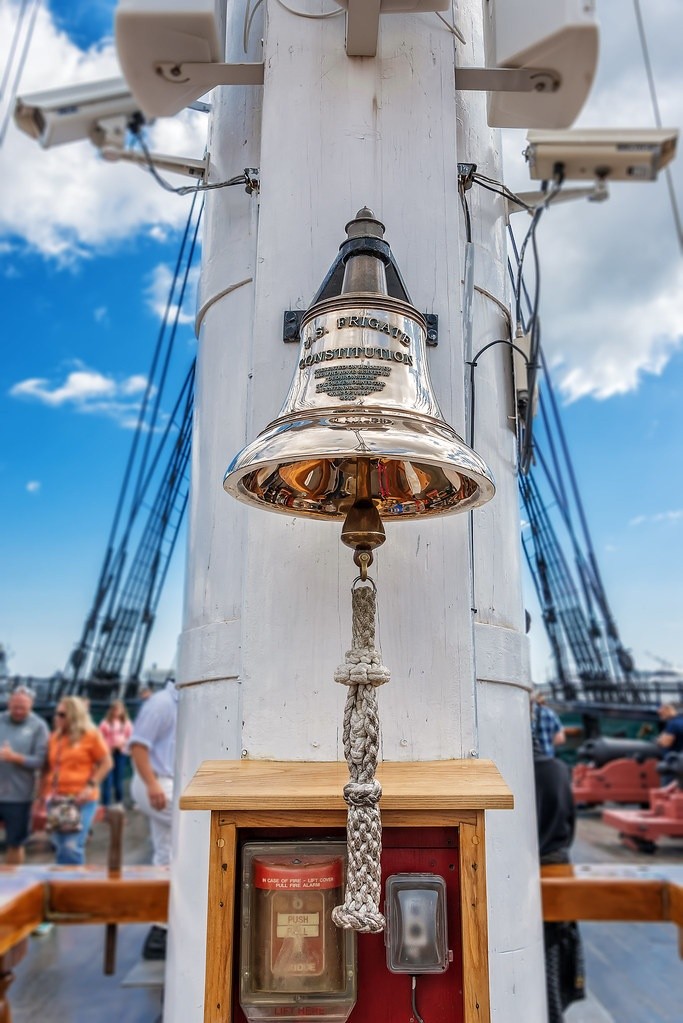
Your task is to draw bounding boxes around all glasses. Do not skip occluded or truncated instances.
[54,711,67,717]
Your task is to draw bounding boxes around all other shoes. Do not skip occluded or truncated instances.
[142,926,167,960]
[32,922,54,935]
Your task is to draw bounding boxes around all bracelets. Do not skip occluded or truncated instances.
[87,779,95,788]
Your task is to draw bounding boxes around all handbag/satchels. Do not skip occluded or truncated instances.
[43,795,82,833]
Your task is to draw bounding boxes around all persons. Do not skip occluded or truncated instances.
[0,686,49,864]
[656,702,683,752]
[127,680,178,961]
[530,682,586,1023]
[99,700,134,811]
[30,695,113,936]
